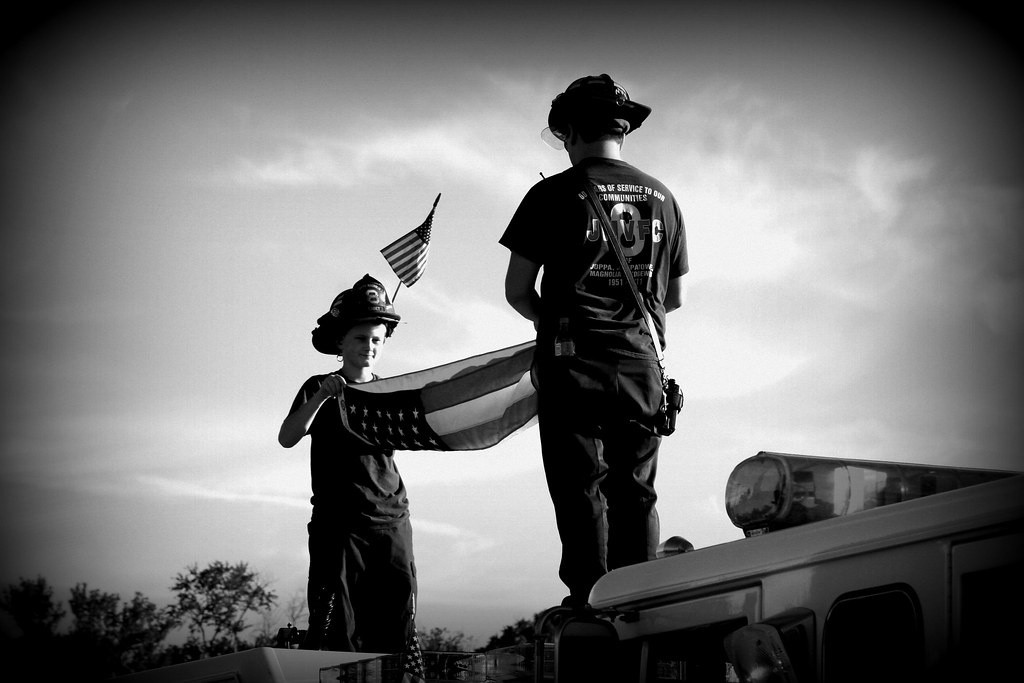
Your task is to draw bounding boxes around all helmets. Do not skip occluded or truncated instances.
[540,72,652,148]
[311,273,401,354]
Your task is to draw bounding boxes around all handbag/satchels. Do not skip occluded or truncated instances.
[657,379,683,435]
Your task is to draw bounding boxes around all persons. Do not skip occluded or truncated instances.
[496,73,693,620]
[278,274,452,652]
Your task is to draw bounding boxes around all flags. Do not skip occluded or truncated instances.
[380,209,434,287]
[333,339,542,454]
[401,592,426,683]
